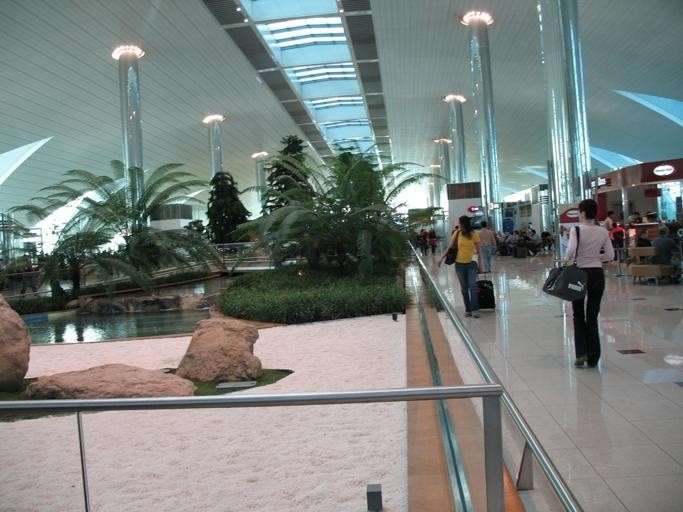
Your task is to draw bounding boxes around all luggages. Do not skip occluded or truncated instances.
[477,272,495,310]
[513,239,528,257]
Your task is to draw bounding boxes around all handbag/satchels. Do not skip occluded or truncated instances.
[542,266,587,301]
[445,247,457,264]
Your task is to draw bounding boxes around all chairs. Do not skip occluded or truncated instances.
[628,247,674,286]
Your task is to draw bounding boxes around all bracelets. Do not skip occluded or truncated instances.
[439,254,444,259]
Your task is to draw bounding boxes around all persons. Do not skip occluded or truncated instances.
[15,256,42,301]
[450,225,459,236]
[411,225,437,256]
[599,209,682,285]
[476,220,496,274]
[558,198,617,368]
[435,214,485,318]
[489,221,554,259]
[569,365,616,511]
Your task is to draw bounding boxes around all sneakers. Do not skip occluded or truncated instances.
[465,313,480,318]
[575,355,588,368]
